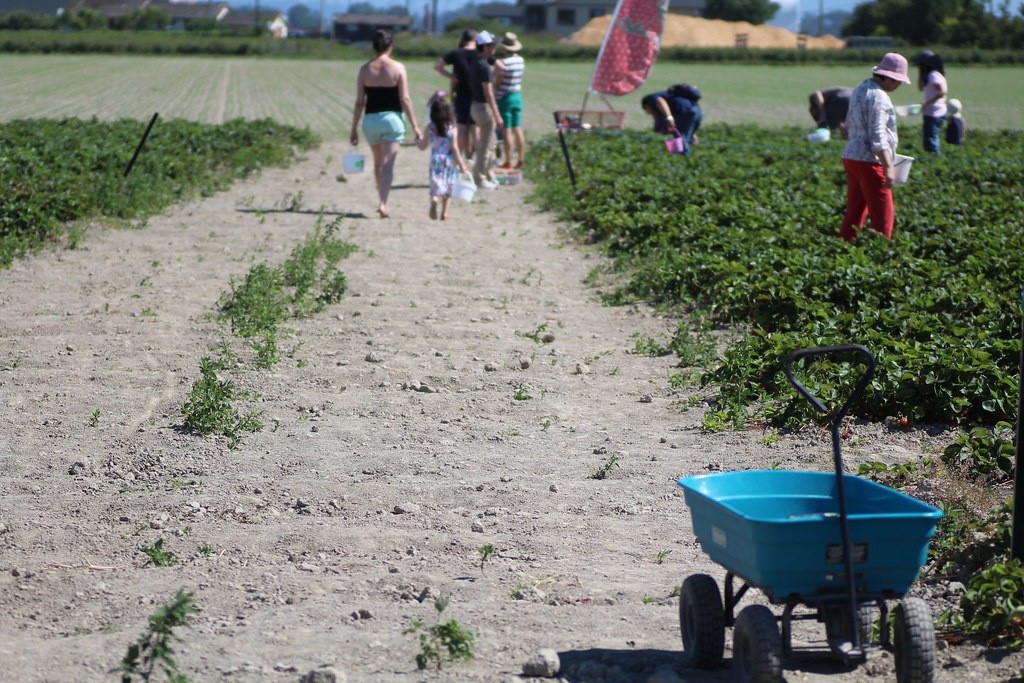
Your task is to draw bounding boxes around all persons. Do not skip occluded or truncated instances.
[945,98,964,145]
[470,30,504,189]
[493,32,526,169]
[415,98,467,220]
[808,86,854,140]
[642,91,702,159]
[350,29,423,218]
[438,31,482,161]
[839,53,911,246]
[912,49,948,155]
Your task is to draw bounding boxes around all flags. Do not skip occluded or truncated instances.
[588,0,668,97]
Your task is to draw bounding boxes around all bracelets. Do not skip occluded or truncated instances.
[667,116,674,121]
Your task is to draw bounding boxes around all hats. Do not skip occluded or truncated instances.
[476,30,494,45]
[948,99,963,118]
[499,33,522,53]
[872,53,913,85]
[428,90,451,101]
[914,50,936,63]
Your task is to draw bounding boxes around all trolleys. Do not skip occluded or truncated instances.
[674,343,944,683]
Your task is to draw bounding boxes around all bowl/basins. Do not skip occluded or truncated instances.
[896,104,922,115]
[496,175,506,184]
[805,133,829,142]
[508,173,522,184]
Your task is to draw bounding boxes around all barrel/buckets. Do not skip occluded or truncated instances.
[666,127,683,152]
[451,171,477,202]
[893,154,914,183]
[343,144,364,173]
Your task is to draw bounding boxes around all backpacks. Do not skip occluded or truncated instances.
[667,83,702,104]
